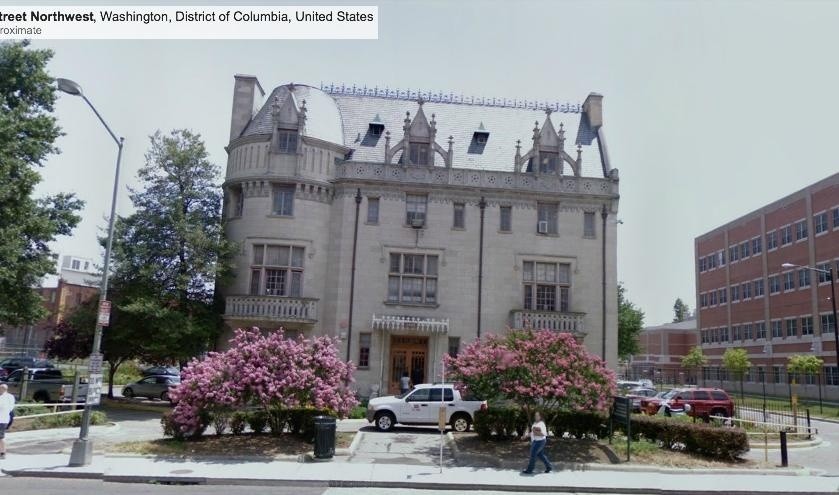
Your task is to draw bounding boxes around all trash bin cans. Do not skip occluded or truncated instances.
[313,416,336,459]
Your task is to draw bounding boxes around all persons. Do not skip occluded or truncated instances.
[398,371,413,394]
[0,382,16,459]
[520,410,554,475]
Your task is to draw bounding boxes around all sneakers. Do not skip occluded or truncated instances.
[523,466,552,474]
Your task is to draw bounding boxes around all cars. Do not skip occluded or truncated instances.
[1,356,183,409]
[366,383,489,432]
[616,380,735,424]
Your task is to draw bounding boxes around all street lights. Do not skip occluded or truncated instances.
[781,262,839,374]
[44,71,128,470]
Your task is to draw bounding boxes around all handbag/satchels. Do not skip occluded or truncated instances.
[7,412,14,429]
